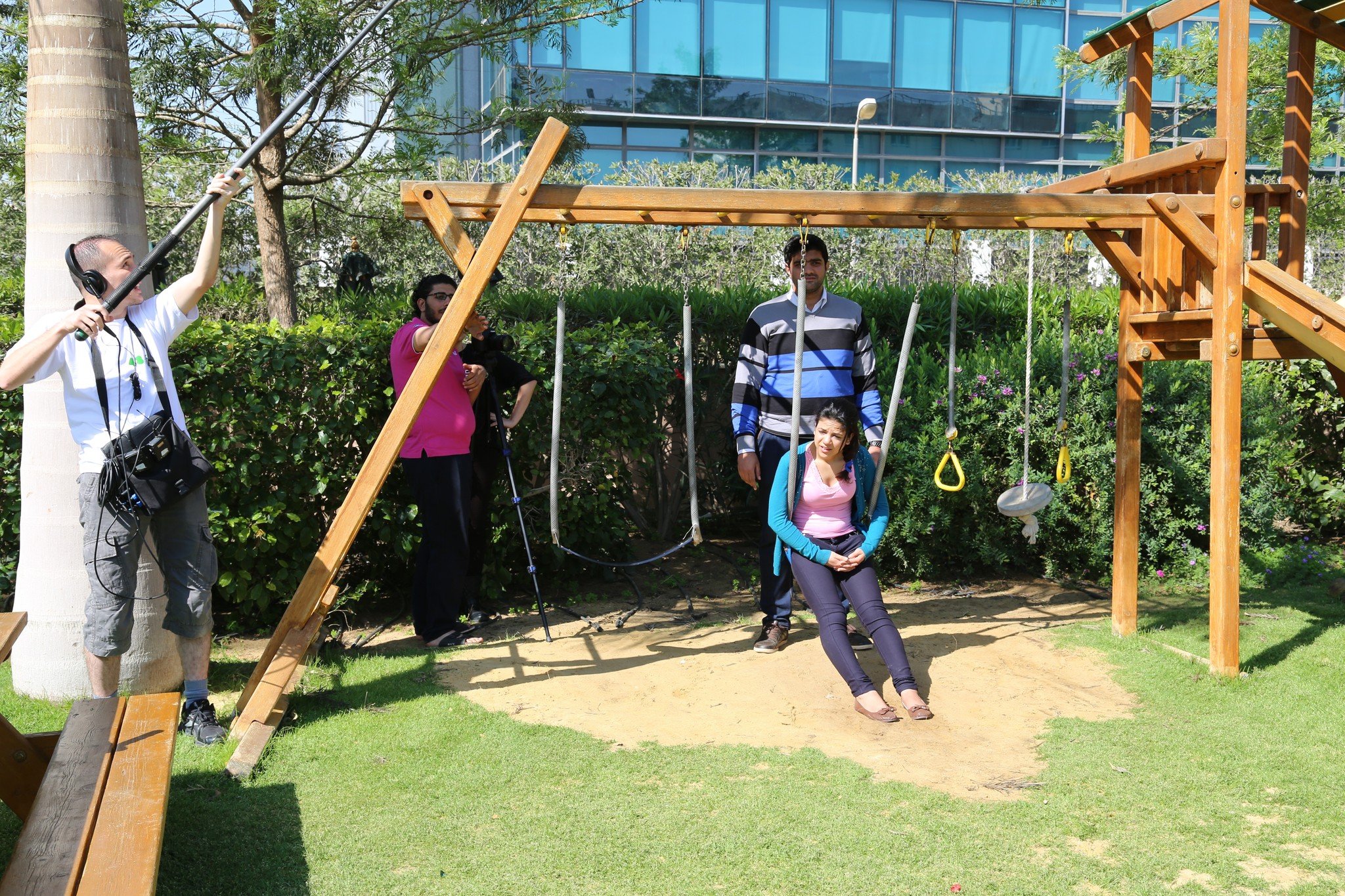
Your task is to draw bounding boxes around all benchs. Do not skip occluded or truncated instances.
[0,692,182,896]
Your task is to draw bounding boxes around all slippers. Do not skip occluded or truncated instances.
[423,631,482,650]
[417,621,475,641]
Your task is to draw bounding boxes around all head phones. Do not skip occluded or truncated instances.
[65,244,107,297]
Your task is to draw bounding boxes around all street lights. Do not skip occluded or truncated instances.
[850,97,880,268]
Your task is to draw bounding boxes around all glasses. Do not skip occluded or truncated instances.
[427,291,454,301]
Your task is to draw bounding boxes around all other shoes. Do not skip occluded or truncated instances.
[855,695,897,722]
[901,698,932,719]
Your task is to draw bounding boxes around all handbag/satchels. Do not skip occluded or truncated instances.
[100,413,215,517]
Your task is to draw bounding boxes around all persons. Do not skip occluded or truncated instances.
[390,273,537,651]
[731,233,883,653]
[0,167,246,747]
[768,398,932,722]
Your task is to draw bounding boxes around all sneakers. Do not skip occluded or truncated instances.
[754,623,789,653]
[178,697,229,747]
[847,623,874,649]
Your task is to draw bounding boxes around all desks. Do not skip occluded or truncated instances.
[0,611,64,824]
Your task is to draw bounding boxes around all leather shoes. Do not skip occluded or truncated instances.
[468,607,488,622]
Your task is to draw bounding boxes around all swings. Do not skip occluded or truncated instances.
[995,229,1055,518]
[549,225,706,568]
[772,226,938,578]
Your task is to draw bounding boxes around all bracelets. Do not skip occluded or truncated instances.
[868,441,881,448]
